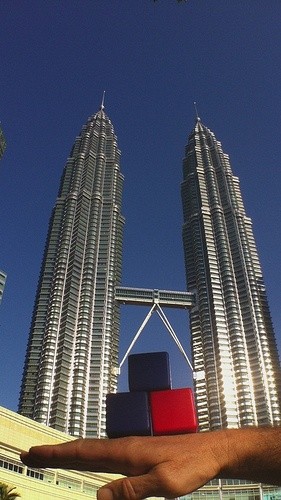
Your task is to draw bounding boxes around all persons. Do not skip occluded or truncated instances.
[19,426,280,500]
